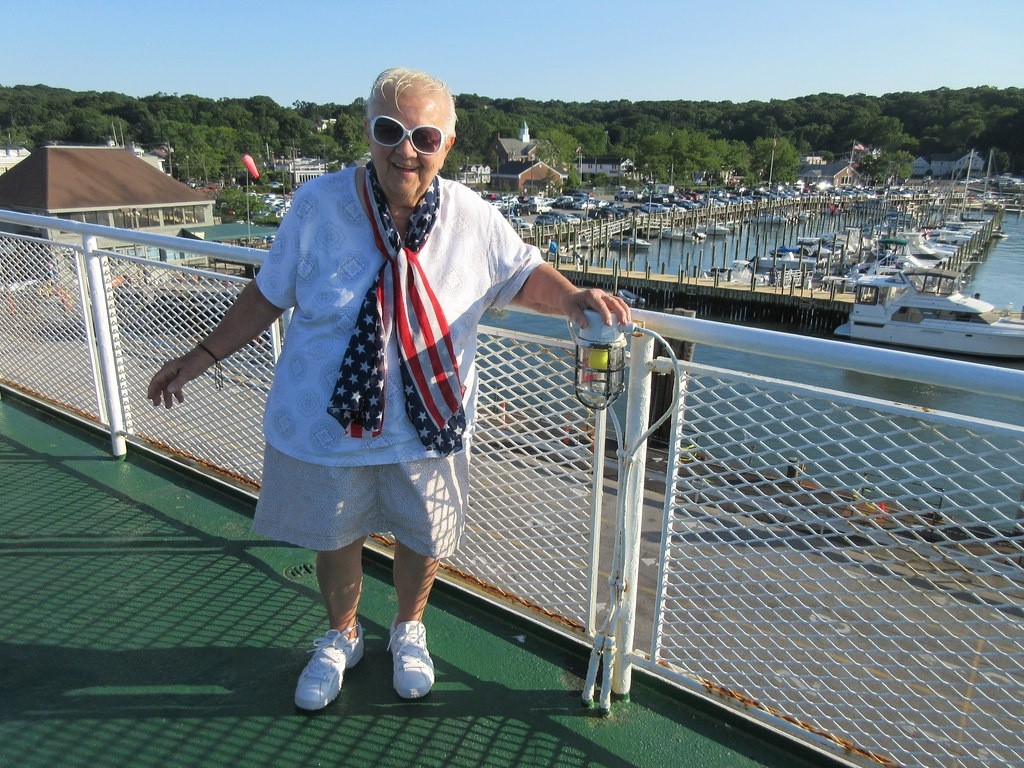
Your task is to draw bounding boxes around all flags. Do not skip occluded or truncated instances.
[854,142,864,150]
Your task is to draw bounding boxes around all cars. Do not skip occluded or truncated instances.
[511,218,534,228]
[271,182,283,186]
[260,193,293,217]
[642,191,739,211]
[642,203,663,213]
[615,191,635,201]
[477,190,640,225]
[737,187,918,204]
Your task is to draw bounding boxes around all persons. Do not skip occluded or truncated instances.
[148,65,631,710]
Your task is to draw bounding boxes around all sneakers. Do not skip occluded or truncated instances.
[294,619,364,710]
[387,614,435,698]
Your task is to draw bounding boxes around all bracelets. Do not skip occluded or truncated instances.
[197,341,224,391]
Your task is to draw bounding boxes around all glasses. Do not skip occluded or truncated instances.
[368,115,452,154]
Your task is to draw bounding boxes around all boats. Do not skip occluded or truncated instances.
[687,220,730,235]
[663,226,707,242]
[832,269,1024,358]
[611,237,652,249]
[821,149,993,291]
[754,211,815,223]
[992,223,1008,238]
[701,237,832,288]
